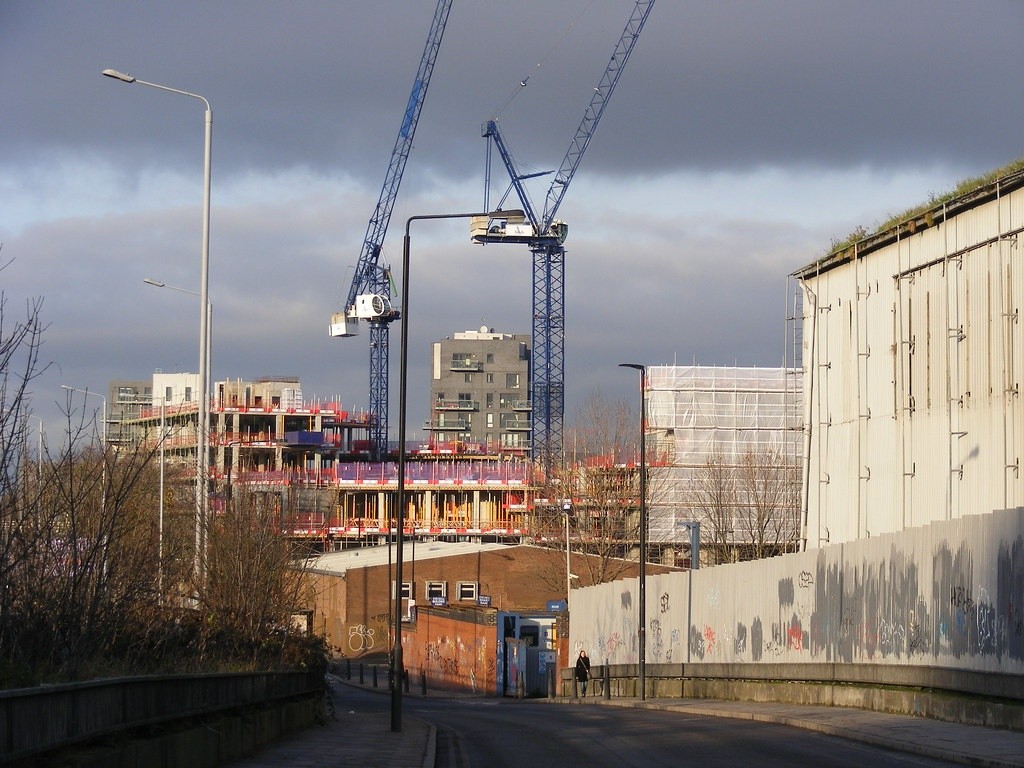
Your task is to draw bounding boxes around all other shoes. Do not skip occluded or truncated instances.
[582,693,585,697]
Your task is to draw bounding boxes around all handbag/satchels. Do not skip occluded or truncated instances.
[587,672,592,680]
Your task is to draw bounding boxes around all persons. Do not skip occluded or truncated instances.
[576,651,590,697]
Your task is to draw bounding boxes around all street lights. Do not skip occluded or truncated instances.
[3,409,43,531]
[61,385,107,595]
[103,69,214,611]
[119,393,164,605]
[143,279,212,610]
[391,209,527,730]
[619,364,646,702]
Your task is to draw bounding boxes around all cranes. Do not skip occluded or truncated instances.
[328,0,453,462]
[470,0,656,464]
